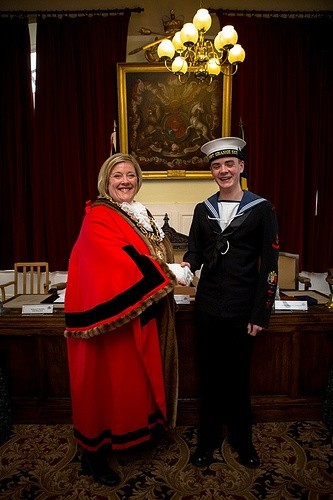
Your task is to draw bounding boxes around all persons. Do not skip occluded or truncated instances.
[64,153,193,487]
[178,137,279,467]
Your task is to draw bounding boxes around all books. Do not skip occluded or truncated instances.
[3,294,65,309]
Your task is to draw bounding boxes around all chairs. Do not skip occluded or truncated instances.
[0,262,52,301]
[278,252,311,290]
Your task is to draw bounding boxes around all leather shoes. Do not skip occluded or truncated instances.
[190,446,220,468]
[82,462,121,487]
[236,442,260,468]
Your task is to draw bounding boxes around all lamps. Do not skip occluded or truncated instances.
[157,0,246,85]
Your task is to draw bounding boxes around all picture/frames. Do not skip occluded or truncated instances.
[116,63,233,180]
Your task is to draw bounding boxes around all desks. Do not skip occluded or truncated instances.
[0,290,333,424]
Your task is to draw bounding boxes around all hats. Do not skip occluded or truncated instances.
[200,136,247,167]
